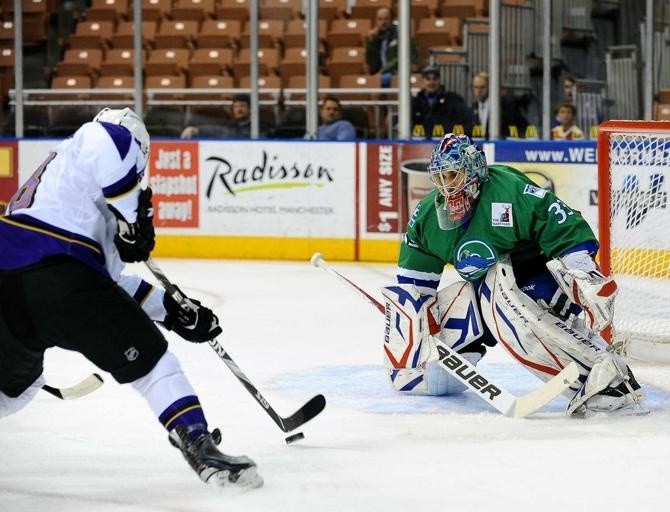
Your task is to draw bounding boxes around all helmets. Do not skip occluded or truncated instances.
[95,107,150,158]
[427,134,489,231]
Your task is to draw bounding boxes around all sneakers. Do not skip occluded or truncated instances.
[586,366,645,410]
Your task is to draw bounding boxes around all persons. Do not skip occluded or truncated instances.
[0,107,264,491]
[550,75,596,141]
[364,7,418,117]
[469,73,529,139]
[382,133,651,422]
[382,63,473,138]
[180,94,270,141]
[303,96,357,141]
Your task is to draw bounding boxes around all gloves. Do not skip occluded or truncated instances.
[105,186,155,264]
[157,285,222,343]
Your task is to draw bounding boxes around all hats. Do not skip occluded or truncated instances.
[422,65,441,76]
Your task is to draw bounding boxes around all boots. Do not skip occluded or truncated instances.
[169,425,257,485]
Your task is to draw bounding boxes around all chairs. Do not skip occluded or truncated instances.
[0,0,59,136]
[655,89,669,124]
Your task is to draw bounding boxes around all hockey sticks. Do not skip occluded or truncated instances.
[310,251,580,420]
[144,256,327,433]
